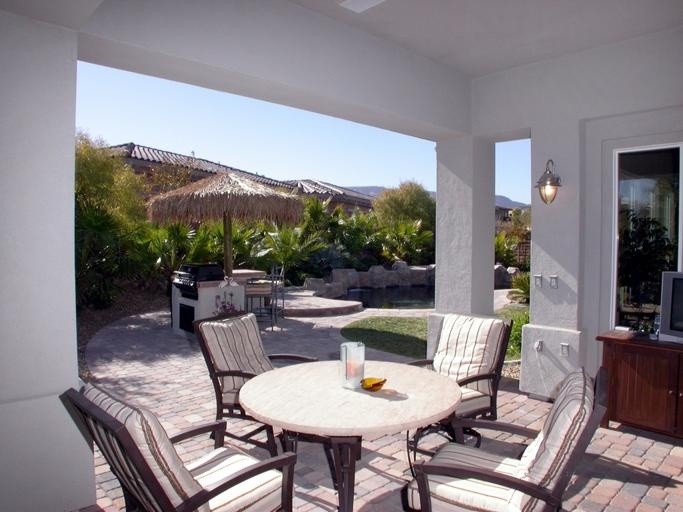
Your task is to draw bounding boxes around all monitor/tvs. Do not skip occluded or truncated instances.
[658,271,683,344]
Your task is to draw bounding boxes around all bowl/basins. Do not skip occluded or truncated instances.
[360,377,386,392]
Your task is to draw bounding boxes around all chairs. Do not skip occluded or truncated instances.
[59,379,295,512]
[400,312,514,455]
[392,360,613,512]
[230,267,288,330]
[190,309,319,472]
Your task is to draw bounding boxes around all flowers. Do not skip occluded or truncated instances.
[209,291,241,317]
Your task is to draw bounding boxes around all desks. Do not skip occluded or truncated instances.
[237,358,463,512]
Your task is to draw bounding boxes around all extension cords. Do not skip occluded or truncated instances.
[649,332,657,341]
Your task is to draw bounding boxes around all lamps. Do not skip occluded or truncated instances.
[532,157,562,205]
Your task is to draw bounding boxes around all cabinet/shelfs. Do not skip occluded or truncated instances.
[595,329,682,444]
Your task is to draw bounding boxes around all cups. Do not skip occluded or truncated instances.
[653,315,664,342]
[338,340,366,390]
